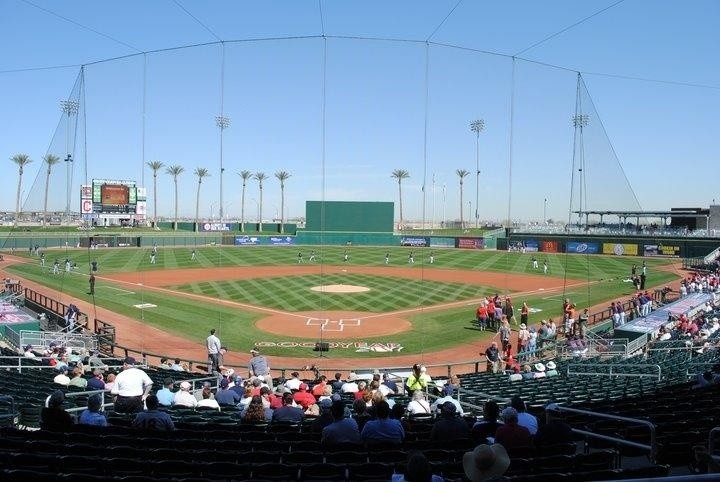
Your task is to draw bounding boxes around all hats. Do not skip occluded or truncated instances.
[546,361,556,369]
[249,350,260,353]
[519,323,527,329]
[437,401,456,413]
[534,363,545,371]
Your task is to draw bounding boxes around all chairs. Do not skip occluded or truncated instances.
[0,340,720,482]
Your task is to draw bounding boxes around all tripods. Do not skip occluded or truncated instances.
[314,324,330,359]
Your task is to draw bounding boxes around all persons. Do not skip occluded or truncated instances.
[392,453,446,480]
[342,371,405,463]
[533,404,577,455]
[153,241,158,253]
[430,252,435,264]
[503,343,517,368]
[691,363,720,388]
[384,252,390,264]
[309,250,317,262]
[470,396,538,459]
[545,361,557,377]
[207,329,221,372]
[191,248,197,261]
[343,254,350,263]
[507,366,523,383]
[486,342,504,373]
[0,222,102,329]
[408,252,416,264]
[533,363,546,378]
[321,400,361,455]
[472,223,720,353]
[522,365,533,380]
[402,363,468,445]
[149,248,157,264]
[296,251,305,264]
[171,347,352,432]
[22,342,174,431]
